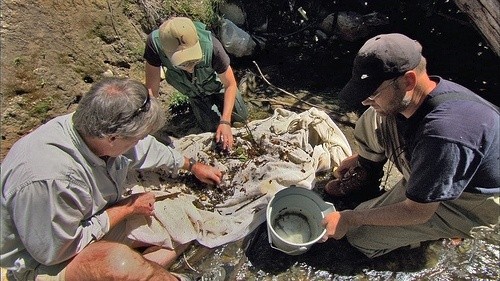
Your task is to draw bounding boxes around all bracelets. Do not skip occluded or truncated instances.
[219,121,231,126]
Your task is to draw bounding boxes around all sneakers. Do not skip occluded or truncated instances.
[325,165,382,200]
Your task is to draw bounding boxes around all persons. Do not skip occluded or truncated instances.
[0,77,223,281]
[320,33,500,259]
[144,17,248,151]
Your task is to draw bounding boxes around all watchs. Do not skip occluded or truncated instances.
[188,158,196,171]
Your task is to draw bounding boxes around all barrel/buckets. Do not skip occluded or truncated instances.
[266,184,336,256]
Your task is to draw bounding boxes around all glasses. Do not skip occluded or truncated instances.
[107,79,153,130]
[364,77,400,100]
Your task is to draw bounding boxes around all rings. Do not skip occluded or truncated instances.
[148,203,152,208]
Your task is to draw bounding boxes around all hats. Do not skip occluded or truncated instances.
[157,17,204,67]
[338,34,423,104]
